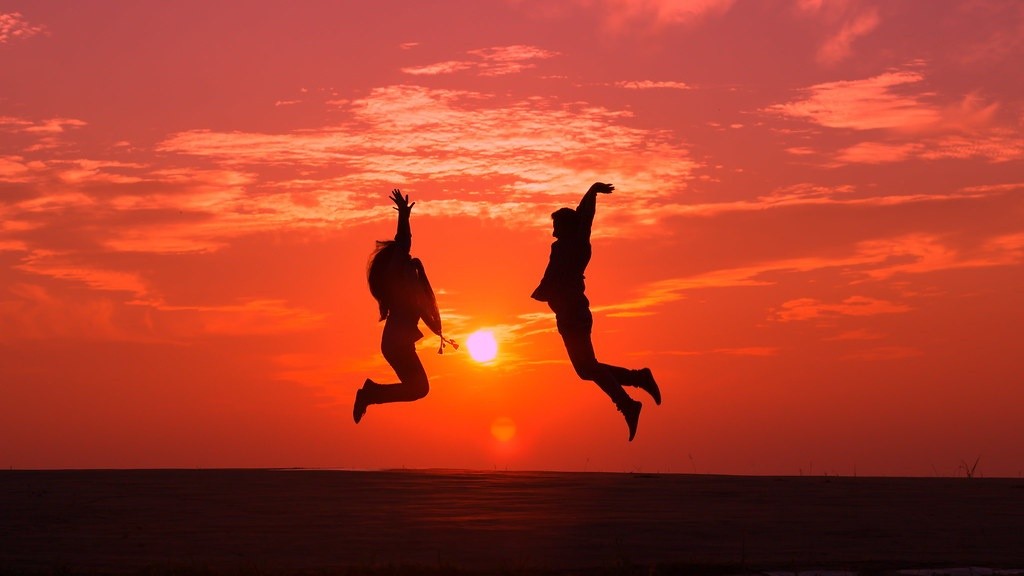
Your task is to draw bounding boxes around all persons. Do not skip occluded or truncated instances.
[529,181,662,442]
[352,187,459,426]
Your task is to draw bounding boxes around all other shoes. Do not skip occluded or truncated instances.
[640,367,662,406]
[625,401,642,442]
[353,378,379,424]
